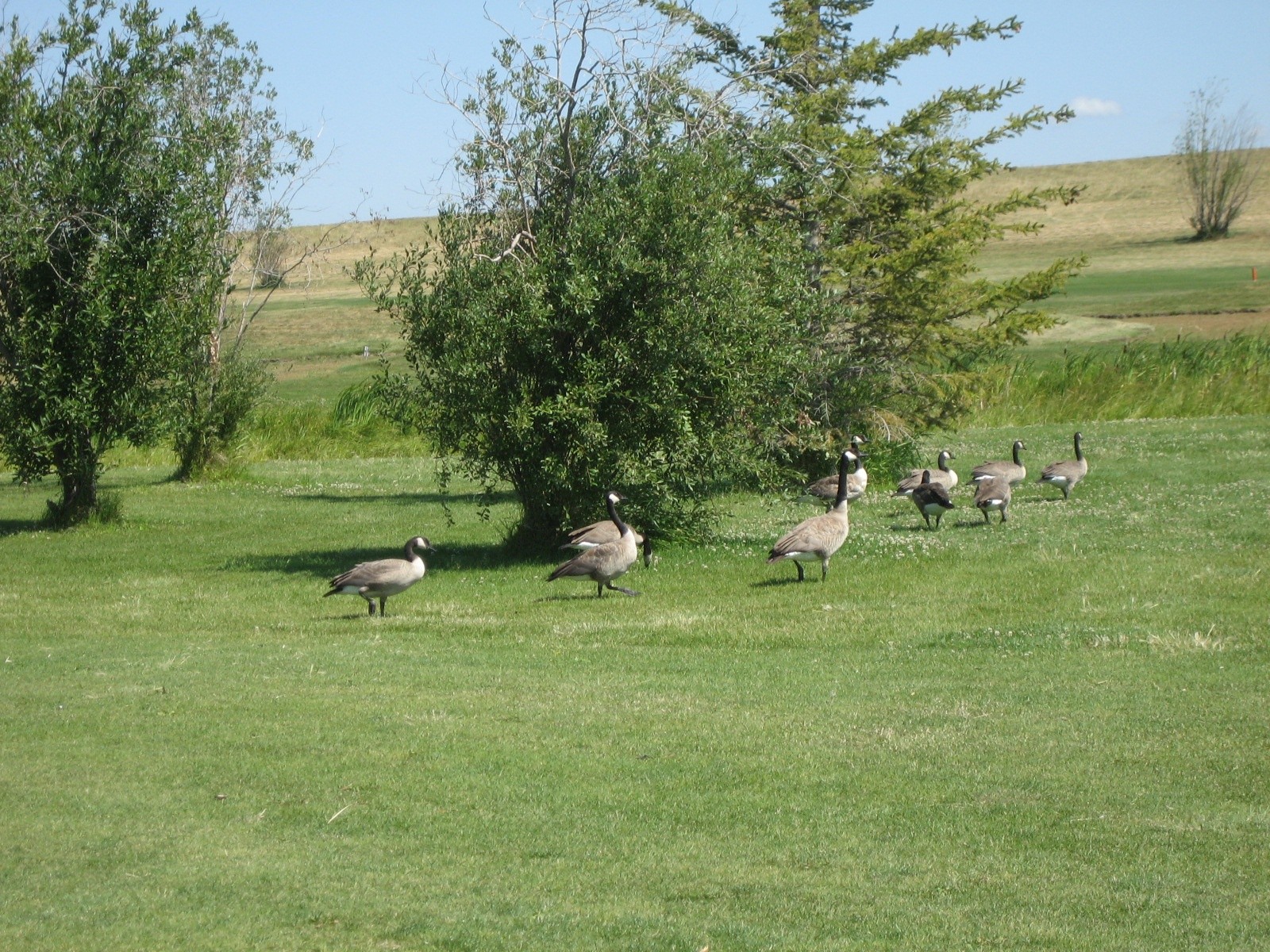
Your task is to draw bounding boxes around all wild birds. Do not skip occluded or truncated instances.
[1035,432,1088,501]
[545,492,653,598]
[323,536,436,619]
[766,449,869,584]
[890,450,959,529]
[804,433,871,513]
[966,441,1028,525]
